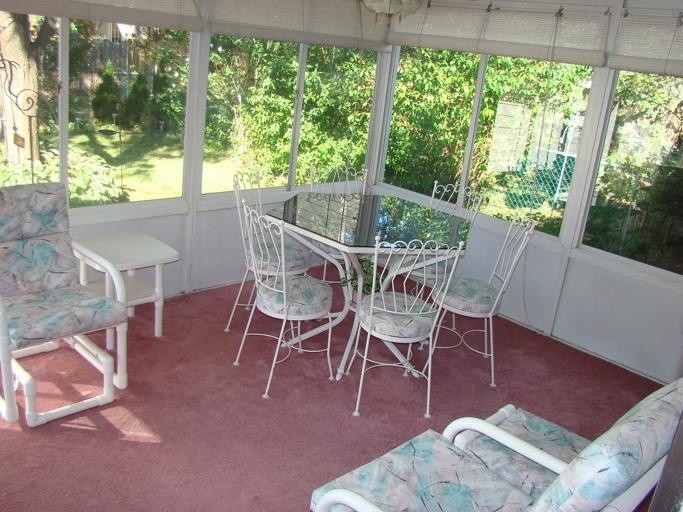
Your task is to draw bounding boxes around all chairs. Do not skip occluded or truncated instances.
[0,182,128,428]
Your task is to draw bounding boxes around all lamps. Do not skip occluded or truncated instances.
[362,0,424,27]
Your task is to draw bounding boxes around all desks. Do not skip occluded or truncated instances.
[73,231,179,350]
